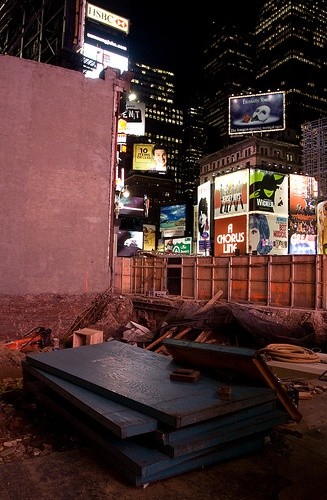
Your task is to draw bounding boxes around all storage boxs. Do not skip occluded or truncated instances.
[73,327,103,348]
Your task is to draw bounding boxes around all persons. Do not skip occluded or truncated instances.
[219,178,246,214]
[150,143,168,171]
